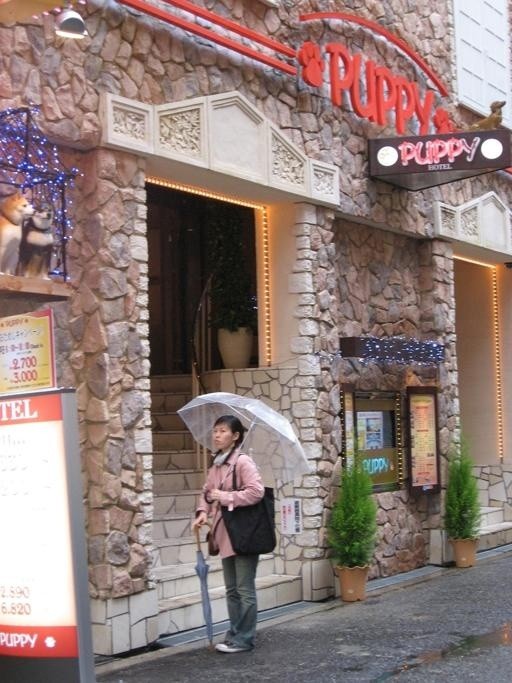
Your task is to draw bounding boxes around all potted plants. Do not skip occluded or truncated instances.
[207,203,259,369]
[326,439,381,603]
[440,428,482,570]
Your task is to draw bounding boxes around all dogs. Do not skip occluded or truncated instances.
[0,188,37,275]
[470,99,505,130]
[15,197,54,281]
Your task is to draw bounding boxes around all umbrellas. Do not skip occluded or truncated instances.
[195,521,214,648]
[176,392,312,504]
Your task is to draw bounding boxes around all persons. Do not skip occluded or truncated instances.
[191,415,265,653]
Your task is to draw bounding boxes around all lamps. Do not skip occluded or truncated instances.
[52,5,91,42]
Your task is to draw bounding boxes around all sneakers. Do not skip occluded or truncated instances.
[214,643,253,653]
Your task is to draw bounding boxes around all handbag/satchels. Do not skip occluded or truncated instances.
[222,485,277,555]
[205,531,220,556]
